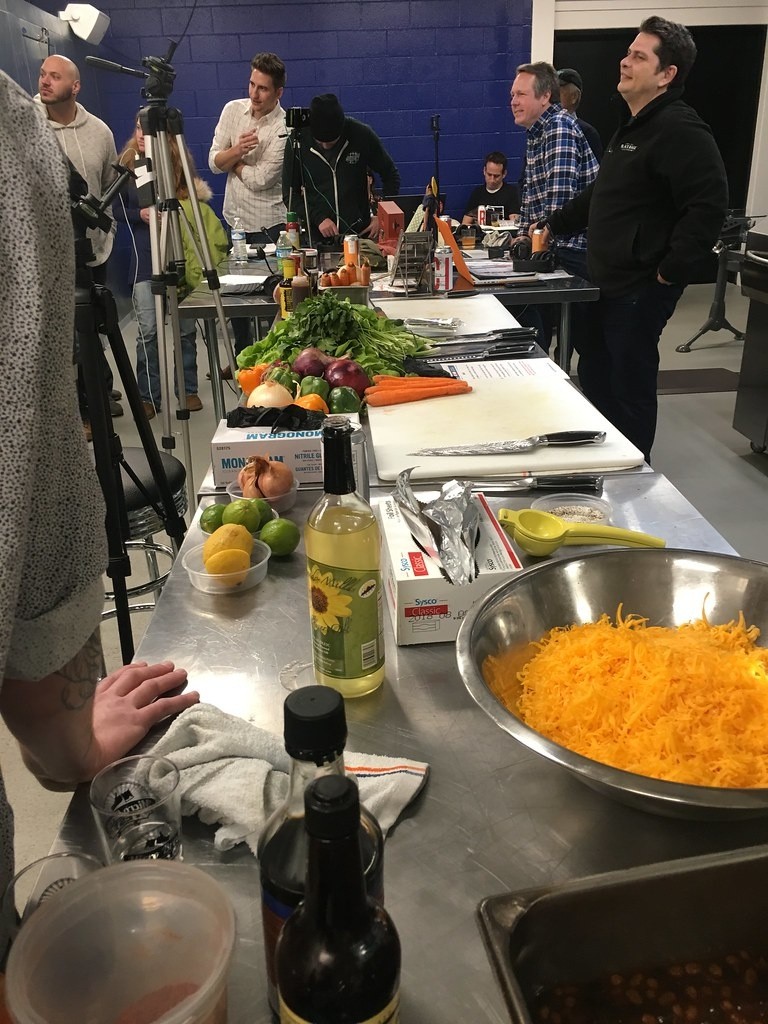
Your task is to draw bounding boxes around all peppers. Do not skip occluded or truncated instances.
[237,364,360,414]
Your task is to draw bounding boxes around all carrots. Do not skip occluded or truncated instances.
[516,592,768,790]
[321,262,371,286]
[364,374,473,406]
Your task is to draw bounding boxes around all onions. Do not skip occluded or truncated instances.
[247,379,293,408]
[292,346,352,376]
[239,455,293,498]
[325,359,373,396]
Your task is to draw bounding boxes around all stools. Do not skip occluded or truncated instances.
[85,448,188,679]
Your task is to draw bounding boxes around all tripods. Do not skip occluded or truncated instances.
[287,127,313,250]
[69,39,243,668]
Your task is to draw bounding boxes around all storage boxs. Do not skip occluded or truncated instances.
[377,492,524,645]
[210,411,360,487]
[378,201,405,246]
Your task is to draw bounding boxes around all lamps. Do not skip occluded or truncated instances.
[58,2,111,46]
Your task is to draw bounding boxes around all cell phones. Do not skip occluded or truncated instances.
[287,107,310,127]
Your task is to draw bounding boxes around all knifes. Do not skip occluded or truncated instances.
[469,476,604,492]
[409,431,608,457]
[417,326,538,364]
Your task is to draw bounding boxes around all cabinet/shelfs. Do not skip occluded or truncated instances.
[733,257,768,455]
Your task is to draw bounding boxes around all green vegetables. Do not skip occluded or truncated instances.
[236,290,441,376]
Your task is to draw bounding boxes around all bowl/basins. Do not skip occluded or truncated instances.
[180,537,272,596]
[531,493,613,525]
[198,509,280,543]
[226,478,300,513]
[454,542,768,820]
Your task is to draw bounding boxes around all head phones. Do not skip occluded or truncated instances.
[509,242,561,273]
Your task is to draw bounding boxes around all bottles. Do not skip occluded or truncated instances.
[276,212,311,320]
[269,775,401,1023]
[305,417,386,696]
[231,218,248,263]
[257,684,386,1023]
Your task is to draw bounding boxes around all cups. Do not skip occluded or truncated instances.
[87,755,184,863]
[5,859,236,1023]
[491,214,500,227]
[3,851,107,939]
[387,255,395,272]
[462,229,475,250]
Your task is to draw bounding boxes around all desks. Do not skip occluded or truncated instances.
[177,246,601,423]
[1,294,768,1023]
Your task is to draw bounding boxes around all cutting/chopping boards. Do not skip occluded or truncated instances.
[366,377,646,482]
[369,294,521,334]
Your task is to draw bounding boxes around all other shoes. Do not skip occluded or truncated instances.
[142,402,155,419]
[185,395,204,412]
[110,388,121,399]
[108,398,123,417]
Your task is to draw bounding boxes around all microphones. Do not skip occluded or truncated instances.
[260,226,276,245]
[256,246,275,275]
[340,217,362,244]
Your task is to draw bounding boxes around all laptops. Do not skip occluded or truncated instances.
[385,193,447,233]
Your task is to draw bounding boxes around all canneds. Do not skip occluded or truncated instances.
[439,215,451,227]
[532,229,548,251]
[477,205,486,225]
[344,234,360,267]
[434,245,454,291]
[288,251,304,276]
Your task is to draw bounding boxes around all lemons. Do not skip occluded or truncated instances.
[259,518,301,556]
[199,498,273,534]
[202,523,253,588]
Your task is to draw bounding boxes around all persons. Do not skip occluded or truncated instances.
[0,70,198,971]
[34,56,625,441]
[529,16,729,465]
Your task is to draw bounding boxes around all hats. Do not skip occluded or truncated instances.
[310,94,344,142]
[558,68,583,90]
